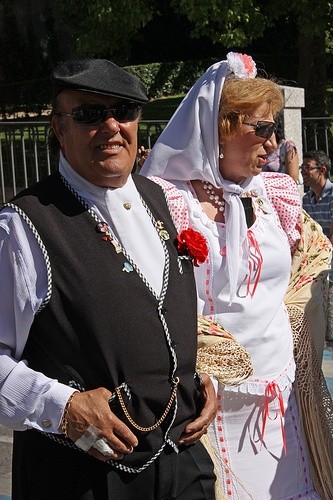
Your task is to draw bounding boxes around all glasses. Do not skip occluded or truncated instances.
[58,102,141,125]
[298,166,322,171]
[241,120,278,139]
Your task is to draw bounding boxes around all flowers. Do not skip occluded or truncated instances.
[226,52,257,80]
[176,227,209,267]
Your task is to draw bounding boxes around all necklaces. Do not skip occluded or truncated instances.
[201,179,225,218]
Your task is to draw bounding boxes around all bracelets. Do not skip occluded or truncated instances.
[60,395,73,438]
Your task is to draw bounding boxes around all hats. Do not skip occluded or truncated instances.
[50,58,149,104]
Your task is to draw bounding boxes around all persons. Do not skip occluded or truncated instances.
[0,60,219,500]
[261,115,299,186]
[146,76,333,500]
[300,151,333,346]
